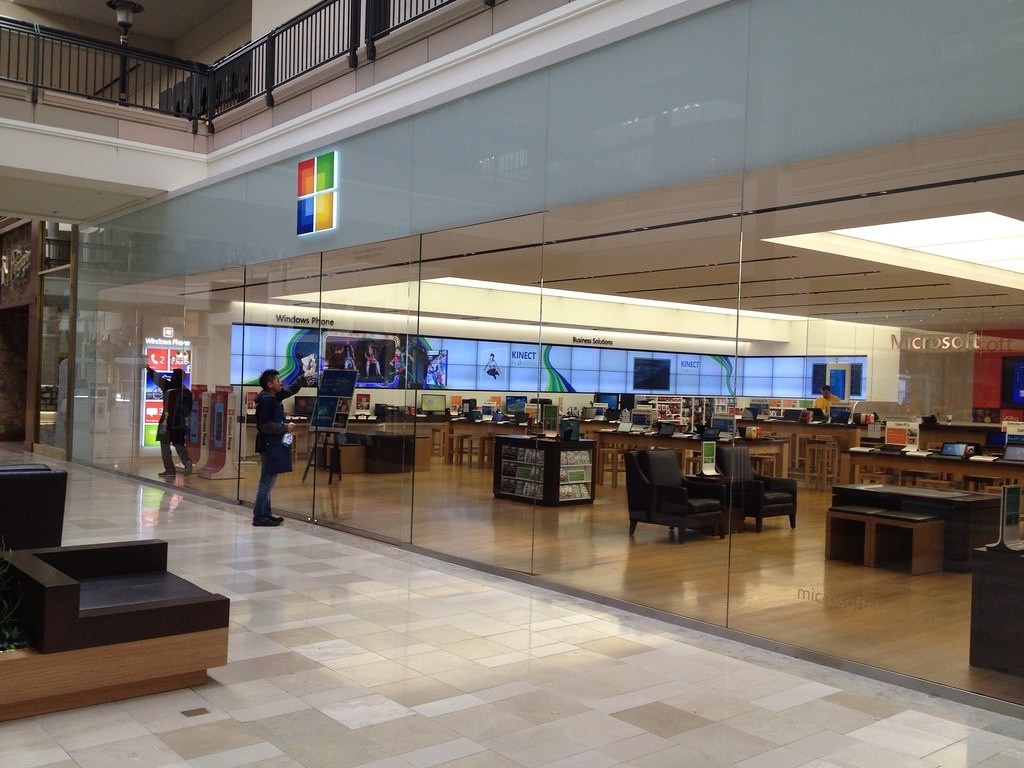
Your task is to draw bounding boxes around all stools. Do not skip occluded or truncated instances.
[826,505,945,574]
[467,434,495,470]
[684,456,701,474]
[290,430,298,463]
[445,433,472,465]
[431,428,446,457]
[762,431,841,491]
[598,447,634,488]
[317,441,366,474]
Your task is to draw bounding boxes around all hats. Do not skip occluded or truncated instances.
[173,369,186,382]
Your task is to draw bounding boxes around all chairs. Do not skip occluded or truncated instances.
[715,443,798,533]
[624,449,727,544]
[0,536,230,654]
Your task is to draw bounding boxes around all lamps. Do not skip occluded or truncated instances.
[107,0,144,29]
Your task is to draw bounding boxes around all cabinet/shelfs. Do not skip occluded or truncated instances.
[493,434,597,506]
[633,395,737,433]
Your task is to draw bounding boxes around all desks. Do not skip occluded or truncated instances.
[684,474,745,535]
[347,422,557,472]
[237,415,312,461]
[580,417,789,506]
[832,483,1002,574]
[847,447,1024,521]
[919,420,1001,433]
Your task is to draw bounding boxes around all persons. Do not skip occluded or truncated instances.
[335,340,416,388]
[146,364,192,477]
[810,385,841,416]
[170,350,191,371]
[253,369,308,526]
[483,353,502,380]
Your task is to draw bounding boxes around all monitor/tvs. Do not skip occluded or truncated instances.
[295,396,316,415]
[596,394,619,416]
[422,394,446,415]
[506,396,527,412]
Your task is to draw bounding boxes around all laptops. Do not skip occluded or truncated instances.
[652,422,677,436]
[929,443,969,459]
[807,407,829,418]
[689,424,721,441]
[979,431,1009,449]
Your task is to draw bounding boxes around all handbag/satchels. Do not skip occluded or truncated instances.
[267,443,293,474]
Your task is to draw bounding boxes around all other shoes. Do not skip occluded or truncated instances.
[158,470,176,477]
[183,460,192,477]
[252,515,284,527]
